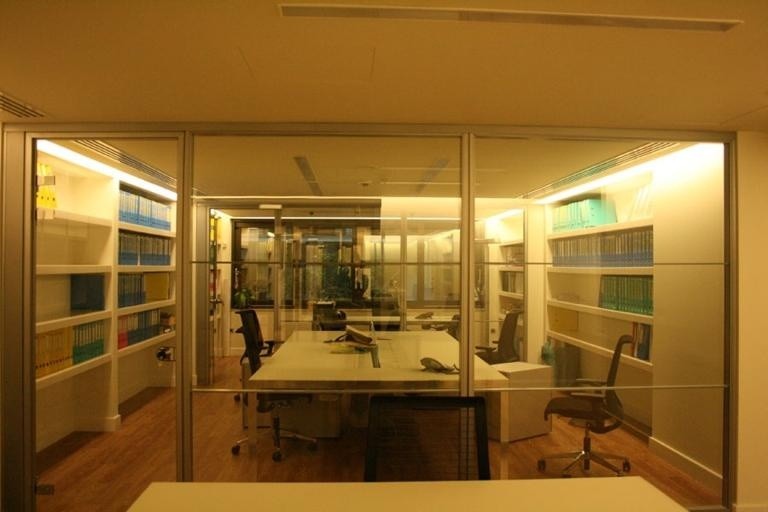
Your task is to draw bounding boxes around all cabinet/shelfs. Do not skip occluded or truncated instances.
[29,140,117,465]
[205,207,233,388]
[116,149,181,426]
[465,208,537,368]
[549,225,653,445]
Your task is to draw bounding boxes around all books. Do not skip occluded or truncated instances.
[119,189,170,231]
[35,320,106,376]
[551,231,654,267]
[71,275,104,308]
[118,274,145,305]
[502,248,524,293]
[118,234,170,265]
[35,165,56,209]
[119,311,161,348]
[598,274,653,315]
[630,322,652,359]
[550,196,616,229]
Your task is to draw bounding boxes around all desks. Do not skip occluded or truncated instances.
[120,476,688,511]
[248,328,507,472]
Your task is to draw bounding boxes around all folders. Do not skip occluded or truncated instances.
[632,323,649,360]
[120,191,172,230]
[552,199,616,230]
[35,320,105,379]
[599,276,653,315]
[118,273,146,308]
[552,232,653,265]
[119,233,172,265]
[119,309,160,349]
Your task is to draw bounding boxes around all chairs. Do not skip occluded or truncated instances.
[313,301,350,328]
[229,308,318,460]
[537,335,644,475]
[362,394,488,482]
[476,309,520,364]
